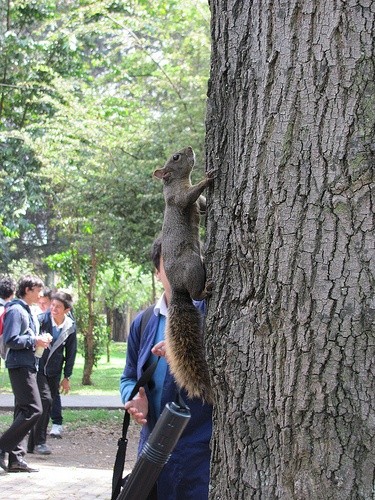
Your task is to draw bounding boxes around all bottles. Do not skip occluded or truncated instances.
[33,333,47,358]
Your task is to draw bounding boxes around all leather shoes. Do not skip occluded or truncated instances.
[0,449,8,472]
[8,459,39,472]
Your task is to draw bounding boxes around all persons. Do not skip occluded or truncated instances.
[0,274,53,472]
[0,275,77,455]
[120,235,212,500]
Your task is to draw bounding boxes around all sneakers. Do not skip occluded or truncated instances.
[27,447,33,453]
[33,443,51,455]
[50,424,63,438]
